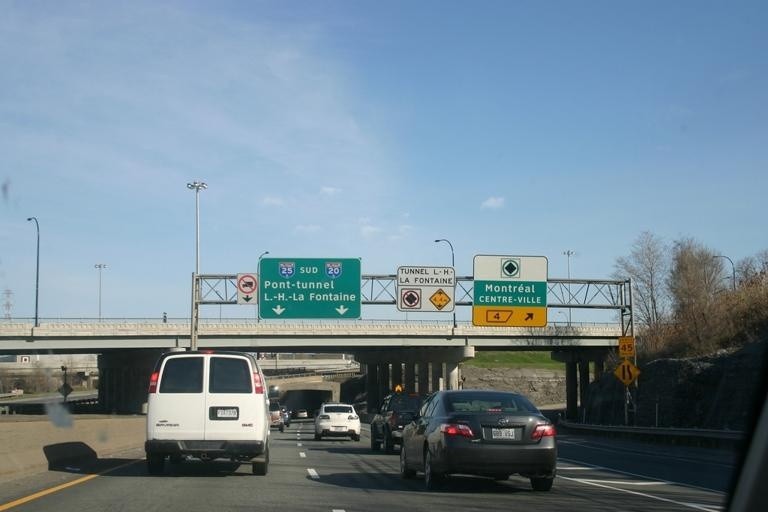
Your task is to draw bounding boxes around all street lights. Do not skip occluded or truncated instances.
[712,255,735,292]
[433,235,456,274]
[186,179,207,276]
[25,218,39,327]
[560,248,574,325]
[556,310,567,322]
[93,264,108,322]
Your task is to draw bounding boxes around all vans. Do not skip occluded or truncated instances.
[144,346,279,476]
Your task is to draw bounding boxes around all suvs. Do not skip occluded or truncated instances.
[268,398,285,434]
[367,391,421,454]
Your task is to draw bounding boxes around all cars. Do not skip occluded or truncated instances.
[397,388,560,491]
[280,406,292,427]
[295,408,308,419]
[312,402,361,445]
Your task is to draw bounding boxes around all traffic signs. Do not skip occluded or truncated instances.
[257,256,364,320]
[470,252,549,327]
